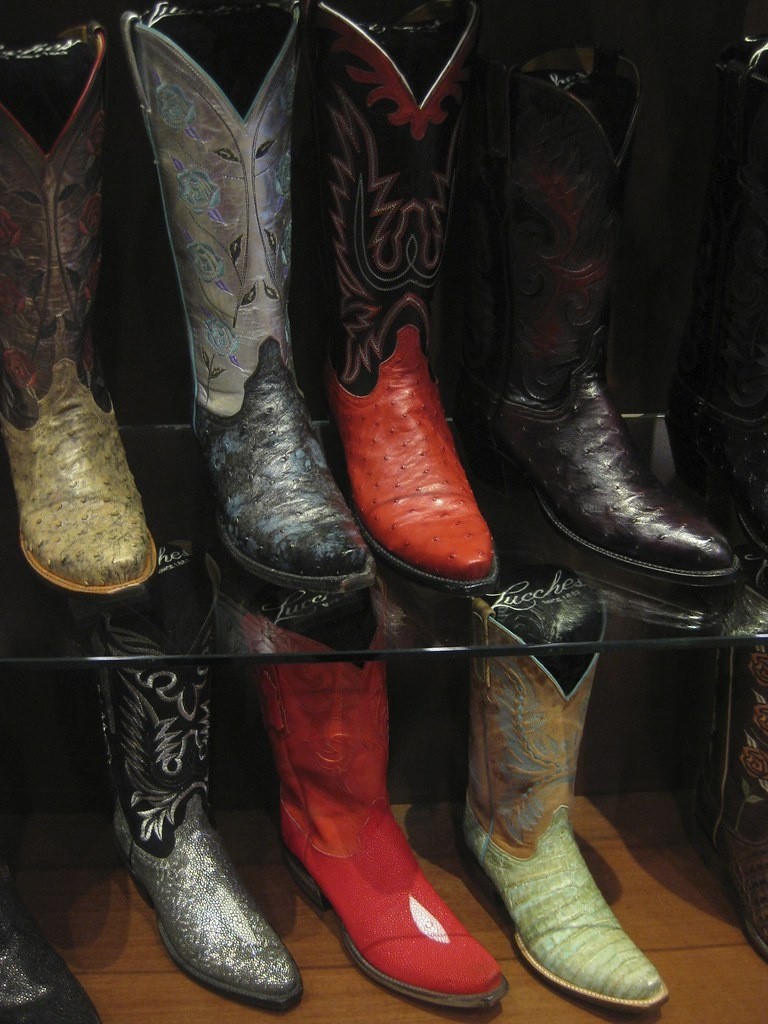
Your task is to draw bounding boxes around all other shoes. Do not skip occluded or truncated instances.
[0,856,102,1024]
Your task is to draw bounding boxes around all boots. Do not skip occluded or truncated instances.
[84,539,305,1011]
[0,19,160,597]
[236,572,510,1009]
[305,0,499,592]
[694,579,768,964]
[472,45,739,586]
[460,563,670,1013]
[118,0,379,594]
[665,31,768,558]
